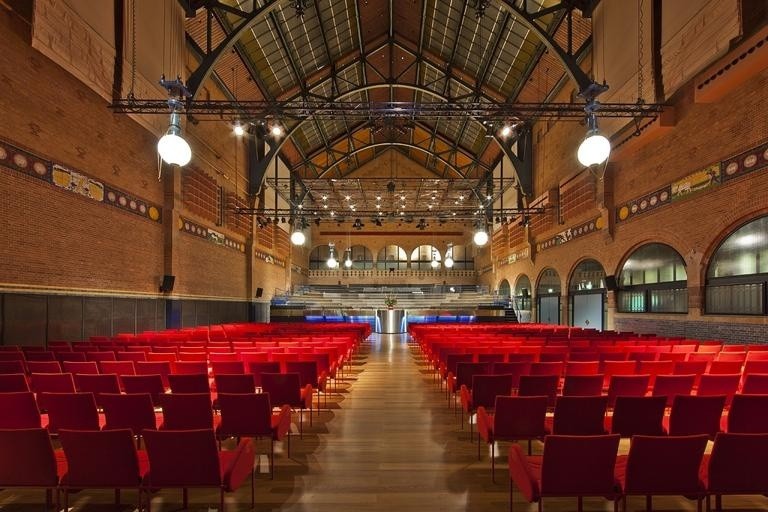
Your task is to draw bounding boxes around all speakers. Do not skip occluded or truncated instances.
[521,288,529,298]
[162,274,175,291]
[604,275,617,291]
[256,287,263,298]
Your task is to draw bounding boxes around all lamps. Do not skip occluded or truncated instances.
[430,253,439,269]
[575,114,614,180]
[444,253,454,268]
[473,219,489,247]
[343,249,354,268]
[154,112,193,183]
[290,221,307,245]
[325,246,337,269]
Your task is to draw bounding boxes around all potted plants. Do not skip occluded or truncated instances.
[385,300,397,310]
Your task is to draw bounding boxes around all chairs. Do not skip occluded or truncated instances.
[0,321,372,512]
[406,321,768,512]
